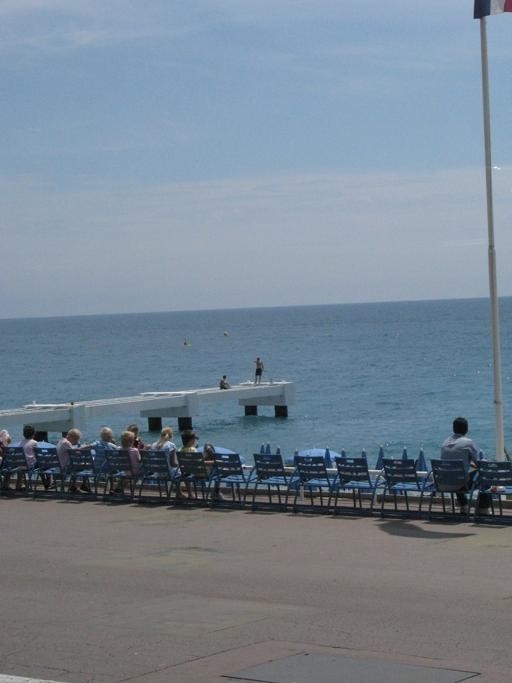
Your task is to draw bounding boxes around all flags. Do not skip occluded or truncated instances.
[474,0,512,19]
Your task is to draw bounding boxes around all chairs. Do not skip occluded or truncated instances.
[212,451,256,509]
[473,460,512,523]
[136,448,180,504]
[251,452,300,513]
[30,446,68,497]
[173,451,218,508]
[66,448,104,500]
[2,446,38,495]
[292,454,342,514]
[428,458,473,520]
[378,457,434,518]
[102,450,144,503]
[334,456,386,515]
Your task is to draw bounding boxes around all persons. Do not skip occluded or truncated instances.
[220,375,232,389]
[440,416,492,515]
[253,357,264,385]
[0,425,216,496]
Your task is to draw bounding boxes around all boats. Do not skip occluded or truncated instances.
[181,341,192,346]
[223,331,229,335]
